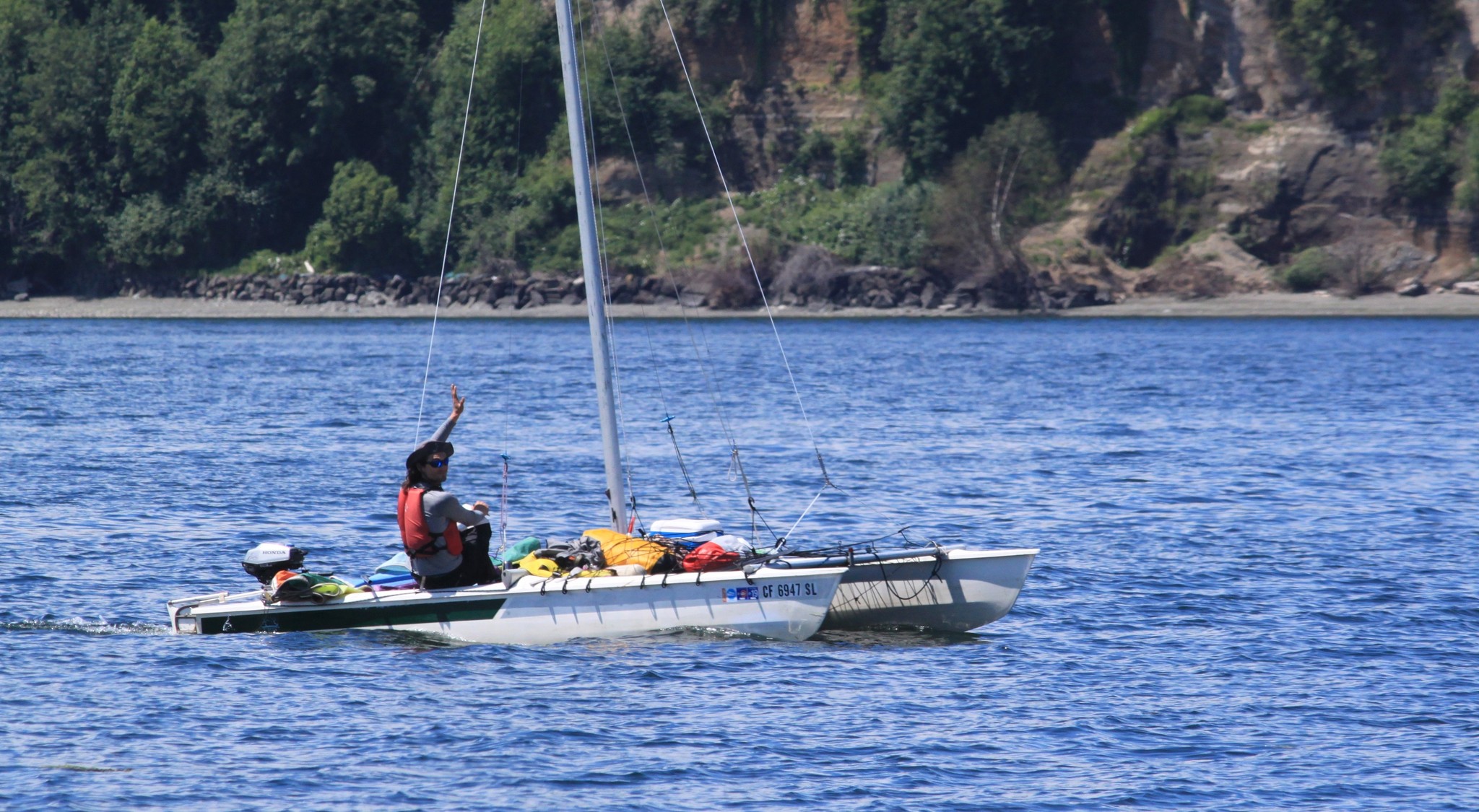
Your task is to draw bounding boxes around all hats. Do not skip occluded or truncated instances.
[406,441,454,470]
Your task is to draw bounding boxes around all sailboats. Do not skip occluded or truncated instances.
[164,1,1039,649]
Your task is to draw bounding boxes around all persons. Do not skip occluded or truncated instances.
[396,385,500,590]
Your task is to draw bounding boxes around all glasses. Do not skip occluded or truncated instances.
[427,458,449,468]
